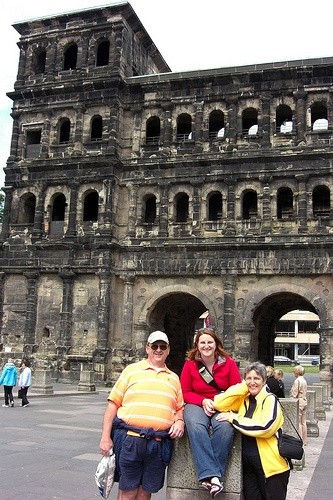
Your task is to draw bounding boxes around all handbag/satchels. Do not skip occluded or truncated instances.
[18,387,26,398]
[262,393,303,460]
[95,448,116,499]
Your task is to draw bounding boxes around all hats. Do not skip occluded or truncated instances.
[8,358,13,363]
[147,330,169,344]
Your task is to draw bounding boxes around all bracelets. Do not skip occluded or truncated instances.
[176,418,185,425]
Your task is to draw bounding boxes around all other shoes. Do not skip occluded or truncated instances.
[2,404,8,408]
[10,401,14,407]
[20,403,30,407]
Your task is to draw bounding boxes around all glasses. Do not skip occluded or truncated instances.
[148,344,170,350]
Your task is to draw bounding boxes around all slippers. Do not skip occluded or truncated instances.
[199,477,224,497]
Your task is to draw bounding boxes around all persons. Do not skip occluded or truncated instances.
[290,365,307,446]
[18,360,32,407]
[213,361,291,500]
[99,331,185,500]
[180,329,242,498]
[265,366,285,398]
[0,357,18,408]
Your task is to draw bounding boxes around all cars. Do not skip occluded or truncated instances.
[273,355,301,367]
